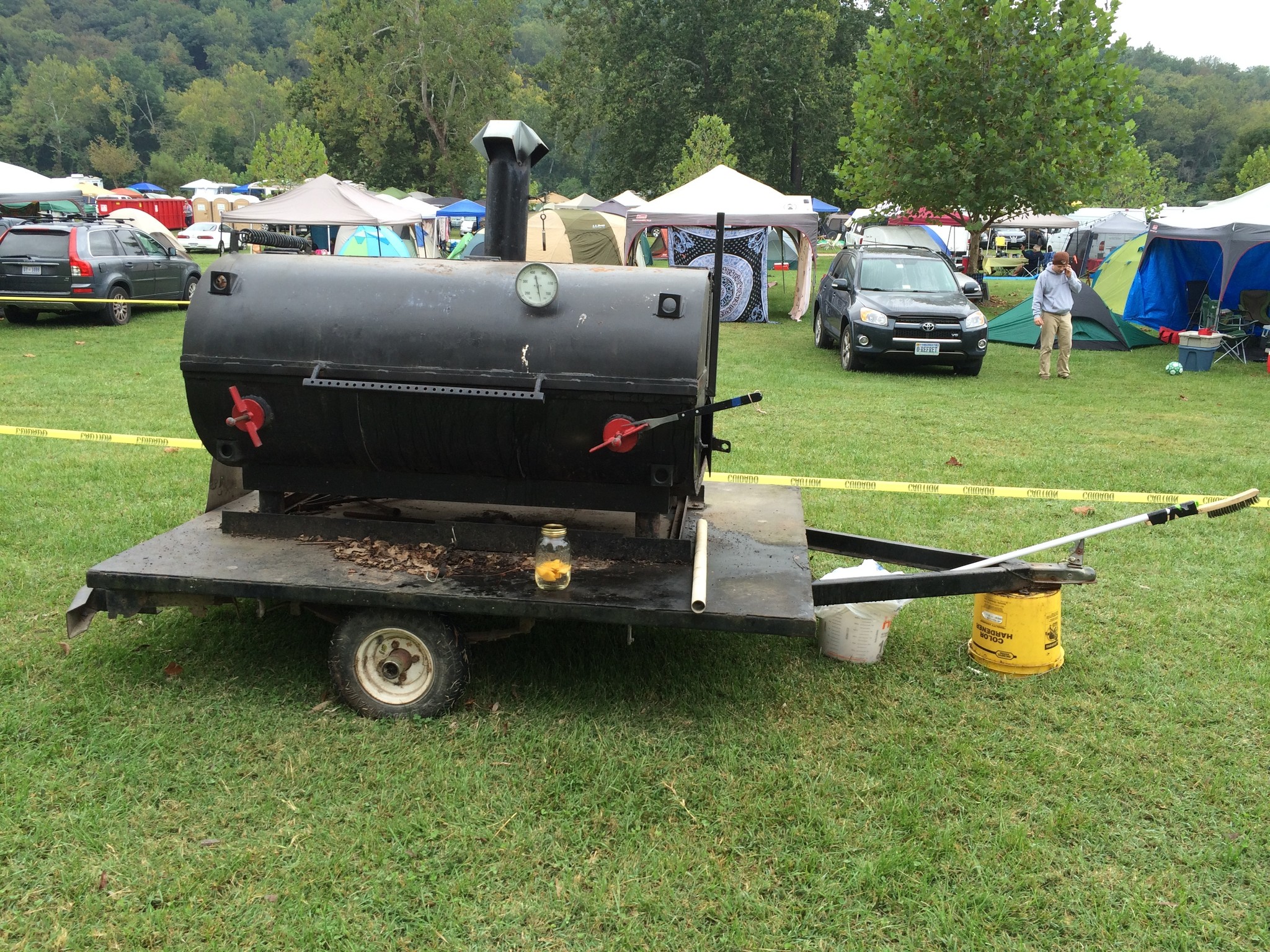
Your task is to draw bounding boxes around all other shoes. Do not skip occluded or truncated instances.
[1058,374,1073,379]
[1041,375,1050,381]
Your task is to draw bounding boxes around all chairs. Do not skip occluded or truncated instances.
[1021,255,1044,278]
[994,236,1008,253]
[1182,279,1270,365]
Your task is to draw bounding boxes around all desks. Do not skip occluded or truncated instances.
[982,256,1029,276]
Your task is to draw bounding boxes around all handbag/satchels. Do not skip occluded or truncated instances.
[1158,326,1185,345]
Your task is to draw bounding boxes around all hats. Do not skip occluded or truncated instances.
[185,200,188,203]
[1053,251,1070,265]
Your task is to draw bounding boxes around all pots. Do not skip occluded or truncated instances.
[1198,325,1216,336]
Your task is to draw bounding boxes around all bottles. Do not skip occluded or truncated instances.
[534,522,572,591]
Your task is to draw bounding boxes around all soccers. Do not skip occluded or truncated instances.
[1165,362,1183,375]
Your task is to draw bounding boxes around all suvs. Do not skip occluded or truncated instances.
[812,243,989,377]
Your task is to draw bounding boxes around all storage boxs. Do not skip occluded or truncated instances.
[1177,330,1225,372]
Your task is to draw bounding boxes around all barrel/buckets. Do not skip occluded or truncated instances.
[958,587,1065,682]
[817,608,893,664]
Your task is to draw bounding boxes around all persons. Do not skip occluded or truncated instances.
[977,248,996,277]
[1030,252,1082,380]
[1009,244,1045,277]
[183,200,193,227]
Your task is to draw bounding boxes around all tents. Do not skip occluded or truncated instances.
[623,164,818,327]
[0,158,1270,348]
[215,174,428,259]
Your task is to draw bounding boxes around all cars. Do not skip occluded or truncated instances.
[177,221,248,254]
[979,226,1075,254]
[459,216,482,237]
[261,223,310,237]
[0,214,203,328]
